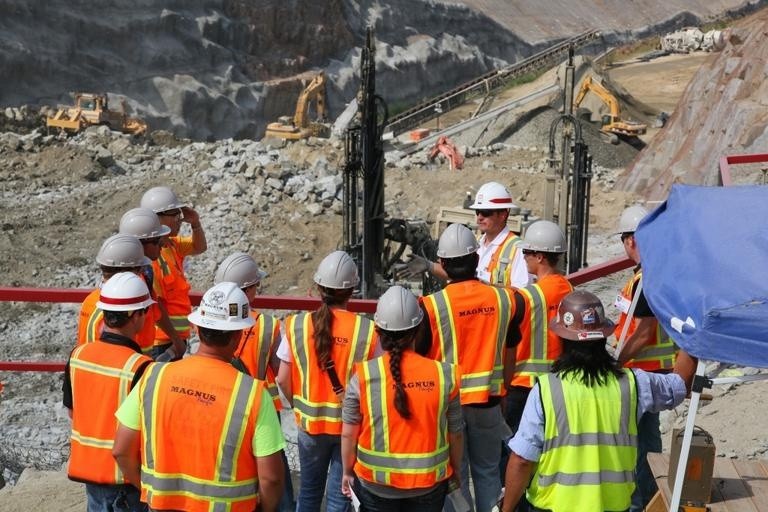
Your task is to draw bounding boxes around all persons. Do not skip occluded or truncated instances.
[213,253,297,512]
[499,290,698,512]
[414,223,519,512]
[63,184,208,512]
[341,285,466,512]
[612,205,681,511]
[500,218,574,486]
[275,250,384,512]
[397,182,535,288]
[113,281,287,512]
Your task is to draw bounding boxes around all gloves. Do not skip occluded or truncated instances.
[397,254,433,278]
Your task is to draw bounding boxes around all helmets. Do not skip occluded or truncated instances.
[313,251,360,289]
[96,271,158,311]
[516,220,569,252]
[188,282,256,331]
[140,187,188,214]
[619,206,648,233]
[96,234,152,267]
[374,286,424,331]
[437,224,480,258]
[119,208,171,240]
[469,182,518,209]
[551,291,615,340]
[214,253,266,289]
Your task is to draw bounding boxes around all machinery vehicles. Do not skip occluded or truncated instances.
[42,91,148,138]
[557,75,647,147]
[260,68,332,143]
[340,23,591,301]
[421,134,466,170]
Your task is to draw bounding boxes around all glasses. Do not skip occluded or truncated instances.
[523,249,546,258]
[245,281,260,293]
[158,211,181,221]
[138,237,161,245]
[621,231,635,244]
[130,307,149,318]
[475,209,506,217]
